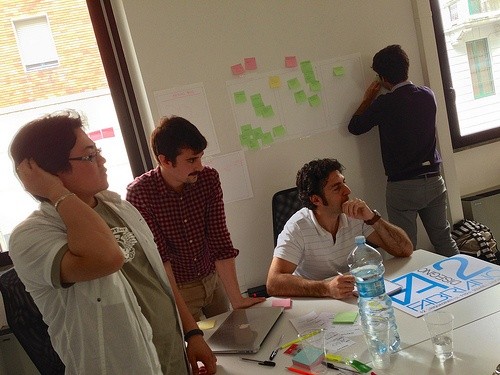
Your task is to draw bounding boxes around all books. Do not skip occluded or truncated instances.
[352,279,402,298]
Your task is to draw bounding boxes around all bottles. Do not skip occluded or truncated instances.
[346,236,401,354]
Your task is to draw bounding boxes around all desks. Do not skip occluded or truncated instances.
[185,249,500,375]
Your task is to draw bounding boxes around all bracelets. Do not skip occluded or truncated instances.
[184,329,204,342]
[54,193,76,211]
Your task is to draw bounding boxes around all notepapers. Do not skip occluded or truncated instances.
[271,298,292,309]
[196,316,218,331]
[292,345,329,372]
[331,310,359,325]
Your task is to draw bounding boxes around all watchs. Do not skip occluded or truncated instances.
[364,209,381,225]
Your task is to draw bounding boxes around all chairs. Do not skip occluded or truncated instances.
[271,187,303,249]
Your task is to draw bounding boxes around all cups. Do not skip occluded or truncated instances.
[422,309,455,360]
[359,316,391,370]
[298,327,327,373]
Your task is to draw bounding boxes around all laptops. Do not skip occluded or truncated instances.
[207,306,285,354]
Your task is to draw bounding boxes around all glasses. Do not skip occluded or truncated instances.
[68,147,102,161]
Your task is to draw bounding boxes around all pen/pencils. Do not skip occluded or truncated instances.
[269,335,284,361]
[336,270,357,286]
[321,361,361,375]
[279,329,322,351]
[240,356,277,368]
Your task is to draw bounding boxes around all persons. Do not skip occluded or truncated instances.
[348,45,459,257]
[8,115,217,375]
[126,116,266,323]
[267,159,413,299]
